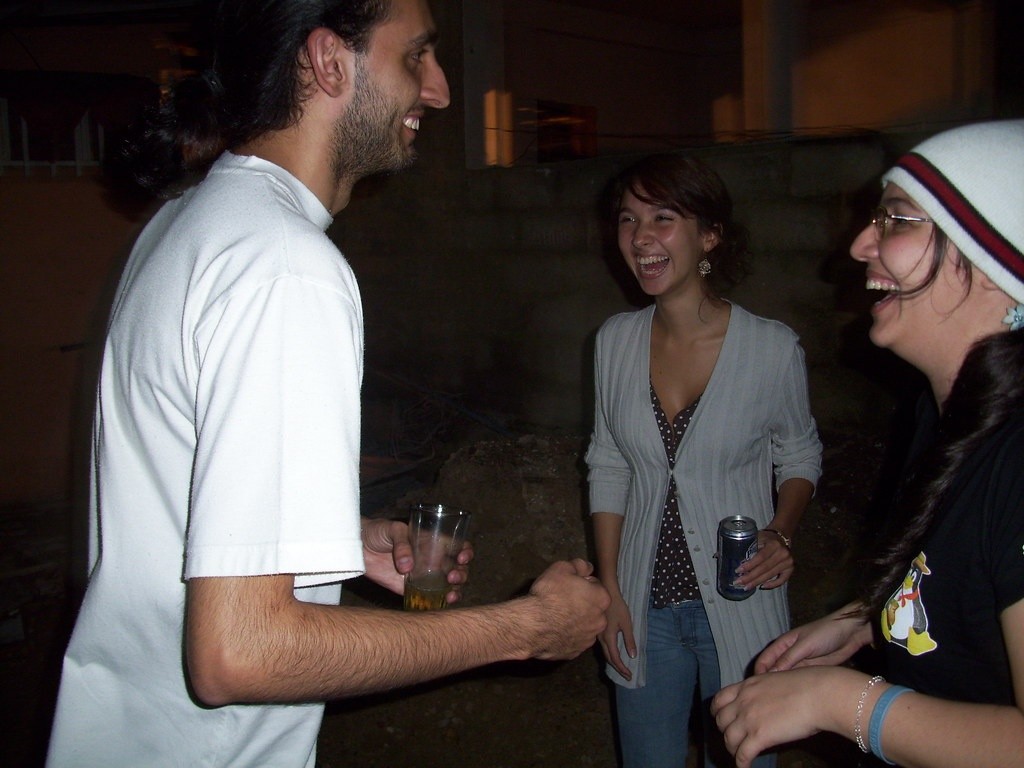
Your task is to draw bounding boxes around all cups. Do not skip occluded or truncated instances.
[404,501,472,612]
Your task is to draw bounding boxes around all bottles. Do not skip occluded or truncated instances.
[711,82,741,143]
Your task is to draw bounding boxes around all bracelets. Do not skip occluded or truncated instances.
[757,527,794,552]
[855,675,916,766]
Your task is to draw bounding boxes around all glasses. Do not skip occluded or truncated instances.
[872,205,935,241]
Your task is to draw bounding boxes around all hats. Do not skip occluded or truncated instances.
[881,119,1024,309]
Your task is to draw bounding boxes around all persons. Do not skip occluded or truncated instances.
[45,0,613,768]
[711,119,1024,768]
[582,151,826,768]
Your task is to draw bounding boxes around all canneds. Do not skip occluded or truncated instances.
[716,515,758,602]
